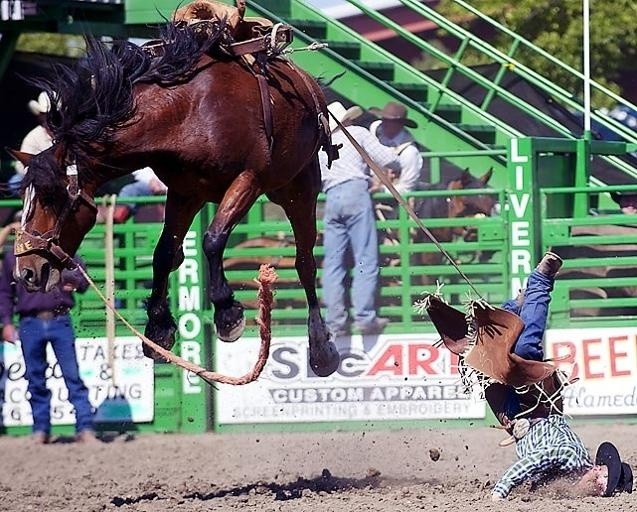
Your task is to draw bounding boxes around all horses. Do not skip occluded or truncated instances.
[221,165,508,326]
[11,0,341,379]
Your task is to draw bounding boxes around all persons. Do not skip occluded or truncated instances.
[1,222,24,247]
[17,87,79,196]
[361,100,423,194]
[0,206,101,442]
[311,97,397,335]
[471,252,634,508]
[97,165,168,223]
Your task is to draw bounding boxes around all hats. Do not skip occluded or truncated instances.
[596,442,632,497]
[28,91,63,115]
[326,101,363,132]
[368,102,417,128]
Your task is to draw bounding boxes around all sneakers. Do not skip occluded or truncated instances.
[360,318,389,333]
[537,251,563,276]
